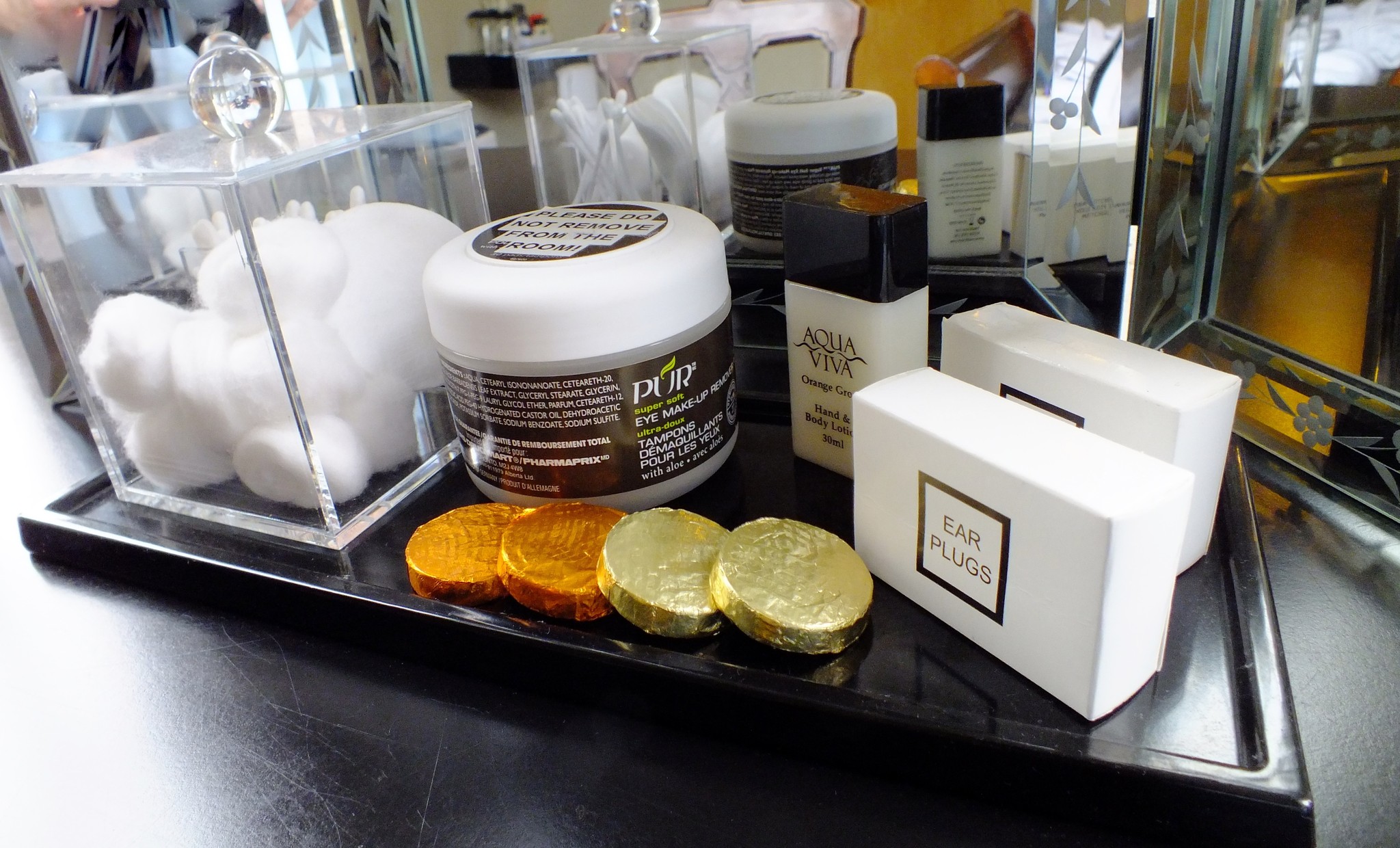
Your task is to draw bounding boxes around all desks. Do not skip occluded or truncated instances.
[0,258,1400,848]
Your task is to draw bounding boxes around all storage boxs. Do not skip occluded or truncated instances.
[938,303,1242,573]
[849,371,1197,721]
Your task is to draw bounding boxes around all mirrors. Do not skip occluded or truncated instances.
[0,0,1400,544]
